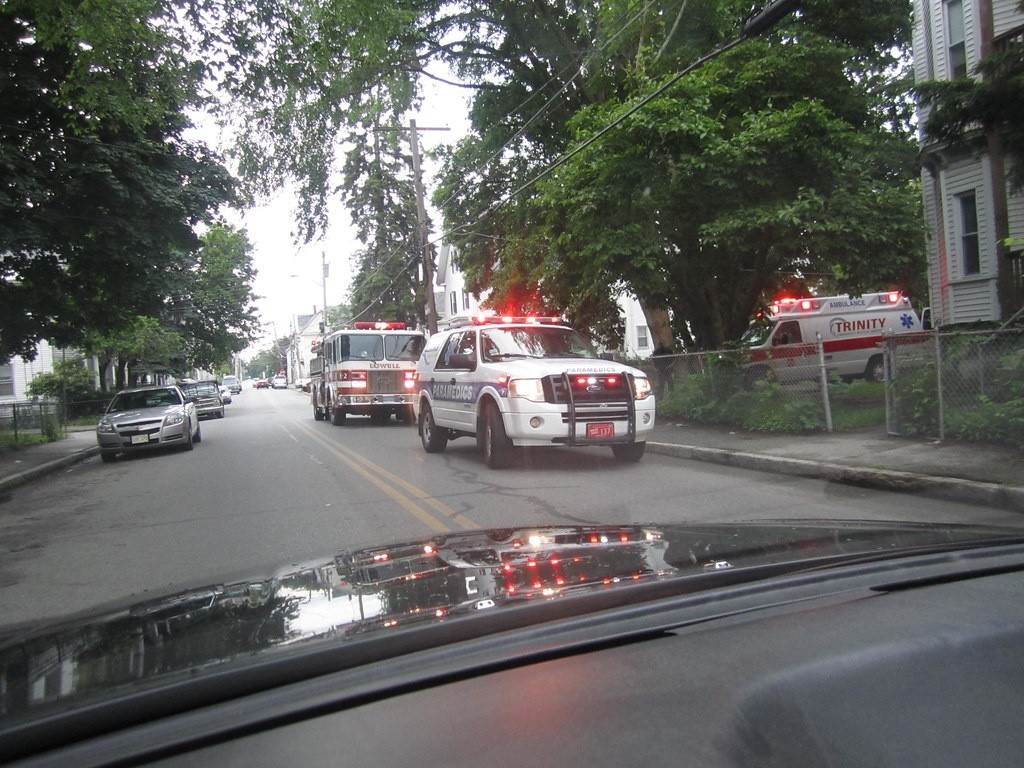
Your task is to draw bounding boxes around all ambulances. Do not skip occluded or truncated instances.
[736,291,927,394]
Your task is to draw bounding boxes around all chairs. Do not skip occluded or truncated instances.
[161,394,178,405]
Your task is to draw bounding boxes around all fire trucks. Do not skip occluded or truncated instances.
[309,321,428,426]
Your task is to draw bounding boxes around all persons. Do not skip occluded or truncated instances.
[653,340,675,401]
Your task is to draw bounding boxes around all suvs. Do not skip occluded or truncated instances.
[412,316,655,470]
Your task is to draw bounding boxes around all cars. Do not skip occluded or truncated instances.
[96,385,201,461]
[252,375,288,390]
[179,375,242,418]
